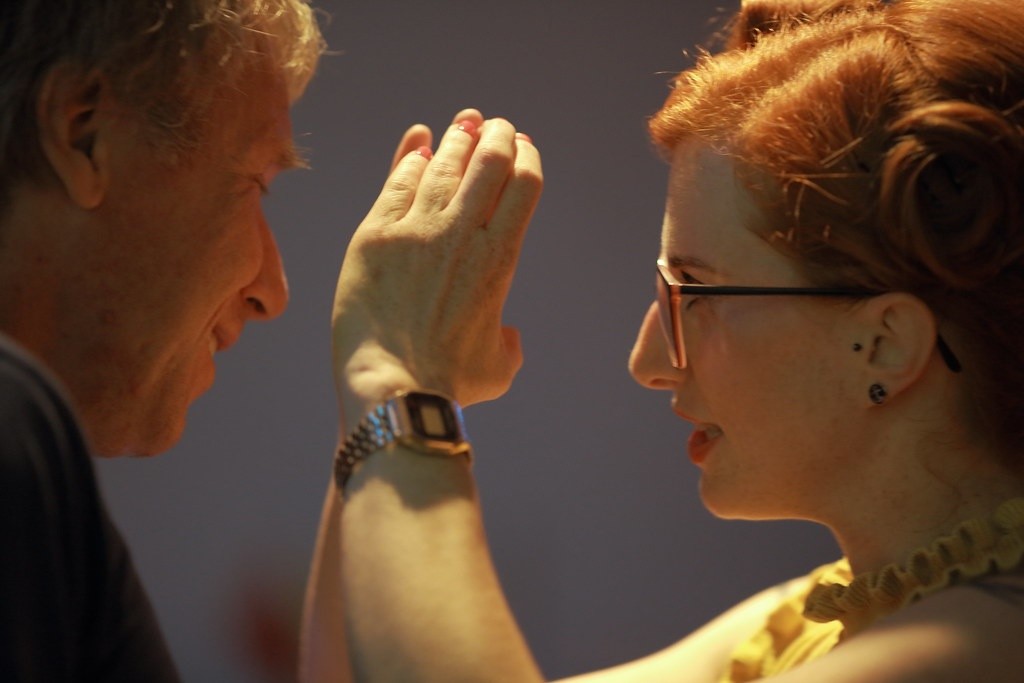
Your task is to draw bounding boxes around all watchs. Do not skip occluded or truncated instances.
[334,388,473,495]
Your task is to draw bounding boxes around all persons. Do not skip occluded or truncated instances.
[298,0,1024,683]
[0,0,320,683]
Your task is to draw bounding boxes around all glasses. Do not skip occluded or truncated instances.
[656,260,964,375]
